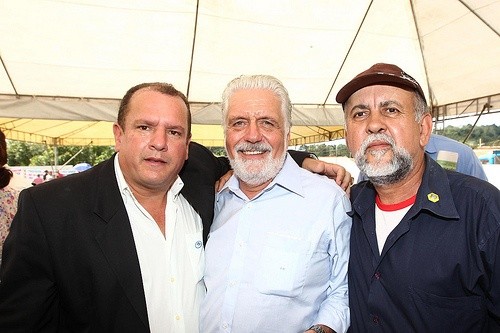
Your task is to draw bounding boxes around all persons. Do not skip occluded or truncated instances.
[30,169,64,186]
[199,74,352,333]
[357,132,487,185]
[0,130,20,268]
[335,62,500,333]
[0,82,355,333]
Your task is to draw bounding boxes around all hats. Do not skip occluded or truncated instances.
[336,63,427,107]
